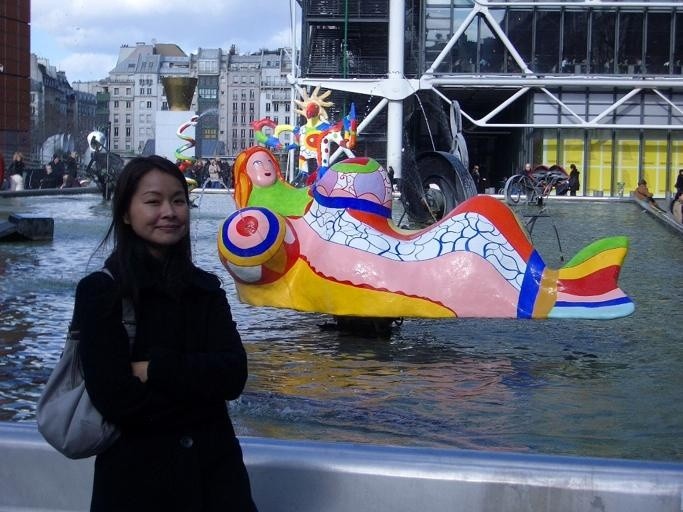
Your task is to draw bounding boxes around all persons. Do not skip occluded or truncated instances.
[635,180,666,213]
[60,150,78,188]
[388,166,395,191]
[452,49,683,76]
[35,164,59,189]
[7,151,24,191]
[472,165,486,194]
[175,157,235,189]
[675,169,683,192]
[669,191,683,224]
[69,154,257,511]
[521,163,533,195]
[346,49,357,79]
[47,154,62,188]
[569,164,580,196]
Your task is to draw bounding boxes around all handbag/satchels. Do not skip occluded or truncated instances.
[34,268,135,460]
